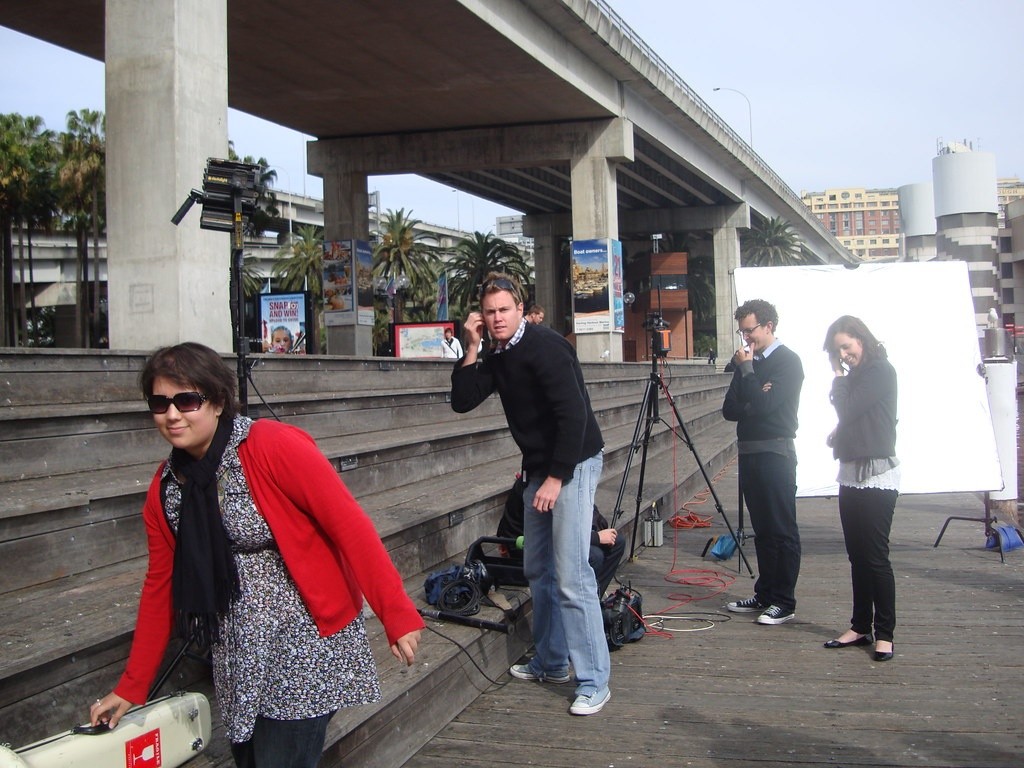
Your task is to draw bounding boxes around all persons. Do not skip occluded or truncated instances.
[450,272,612,716]
[708,347,716,369]
[264,326,305,354]
[440,327,463,358]
[497,458,626,600]
[721,298,806,626]
[90,341,425,768]
[525,303,546,325]
[822,315,901,661]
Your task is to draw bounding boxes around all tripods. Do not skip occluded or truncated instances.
[610,317,756,578]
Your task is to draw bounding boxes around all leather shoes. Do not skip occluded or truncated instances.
[874,642,894,662]
[824,633,874,648]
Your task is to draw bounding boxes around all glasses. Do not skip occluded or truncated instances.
[737,325,761,335]
[146,392,209,414]
[481,279,521,301]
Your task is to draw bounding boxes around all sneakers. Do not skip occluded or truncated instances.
[757,605,796,625]
[511,664,571,684]
[727,597,767,612]
[570,688,612,715]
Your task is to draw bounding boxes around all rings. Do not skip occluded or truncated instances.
[96,698,101,706]
